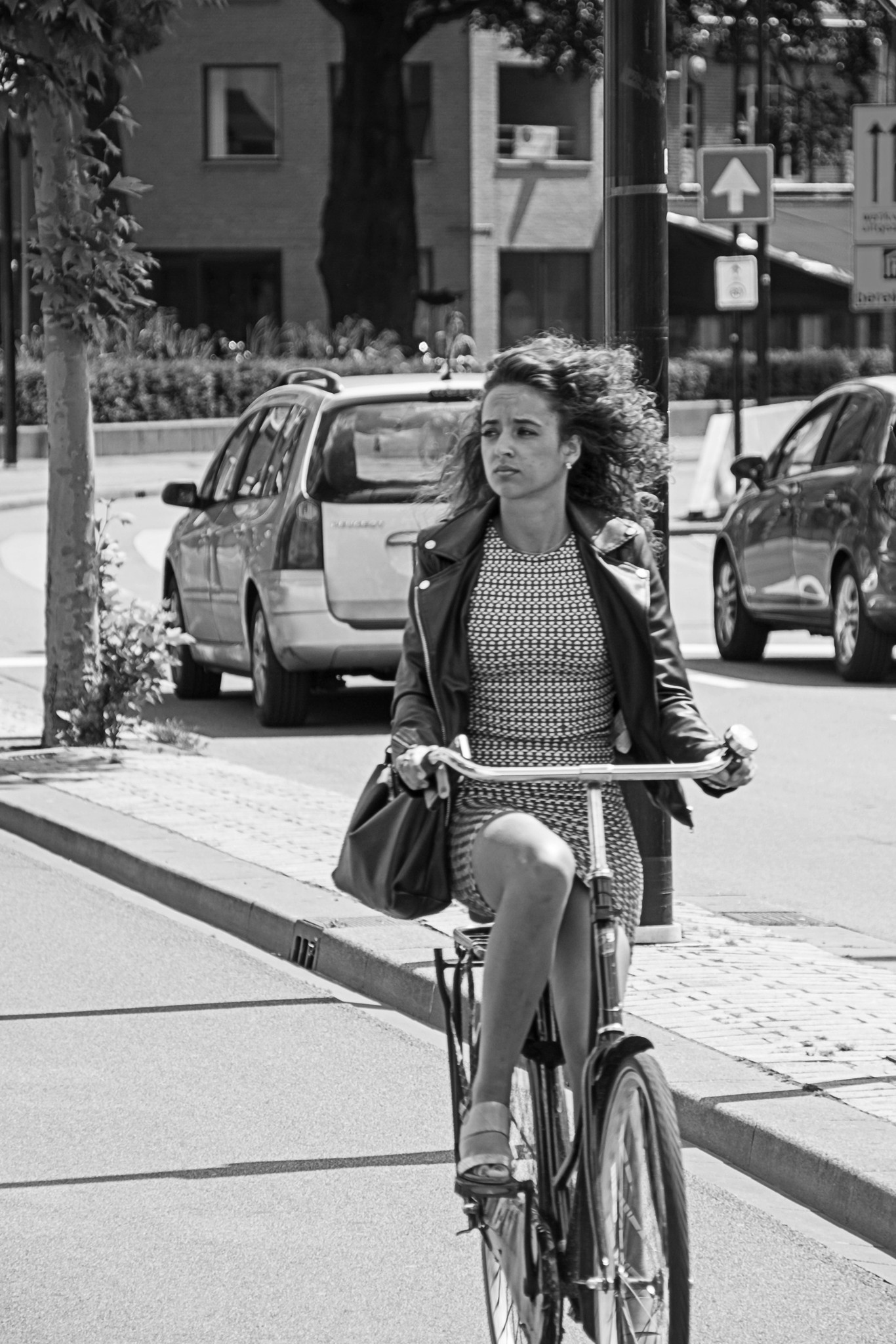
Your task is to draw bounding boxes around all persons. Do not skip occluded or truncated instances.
[429,311,479,365]
[392,332,755,1344]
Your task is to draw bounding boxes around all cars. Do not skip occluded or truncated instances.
[712,373,896,683]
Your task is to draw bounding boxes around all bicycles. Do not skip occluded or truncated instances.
[393,722,759,1344]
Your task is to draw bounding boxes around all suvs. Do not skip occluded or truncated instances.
[160,365,504,731]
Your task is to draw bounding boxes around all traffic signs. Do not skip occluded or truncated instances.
[852,103,896,245]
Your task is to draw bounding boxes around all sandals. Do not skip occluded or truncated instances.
[457,1095,513,1183]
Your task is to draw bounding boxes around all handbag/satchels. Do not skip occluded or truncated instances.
[331,763,453,920]
